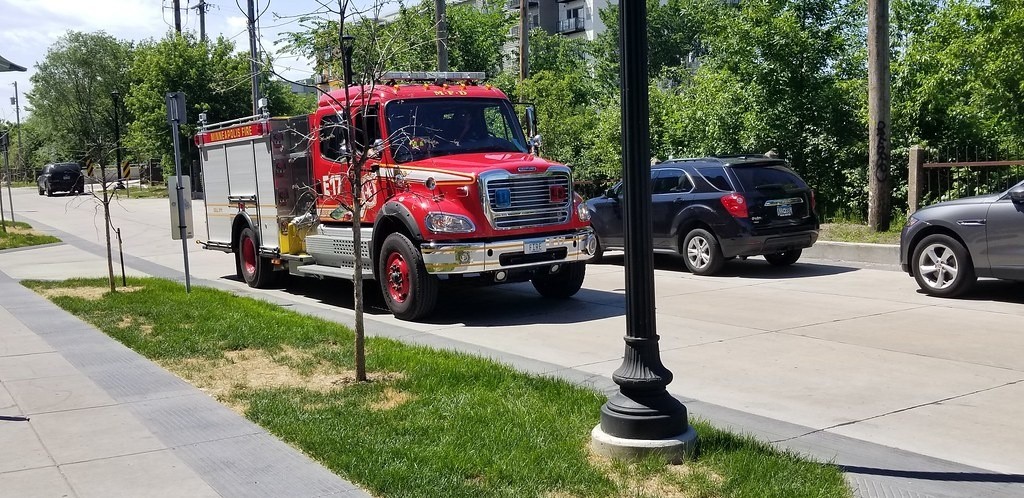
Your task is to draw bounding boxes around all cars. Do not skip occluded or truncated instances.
[900,179,1024,297]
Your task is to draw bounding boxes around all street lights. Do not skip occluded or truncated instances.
[343,30,355,86]
[111,89,125,189]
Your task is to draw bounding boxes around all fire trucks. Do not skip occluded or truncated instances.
[193,69,596,321]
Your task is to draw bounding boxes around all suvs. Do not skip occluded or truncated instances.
[36,163,84,196]
[581,154,820,276]
